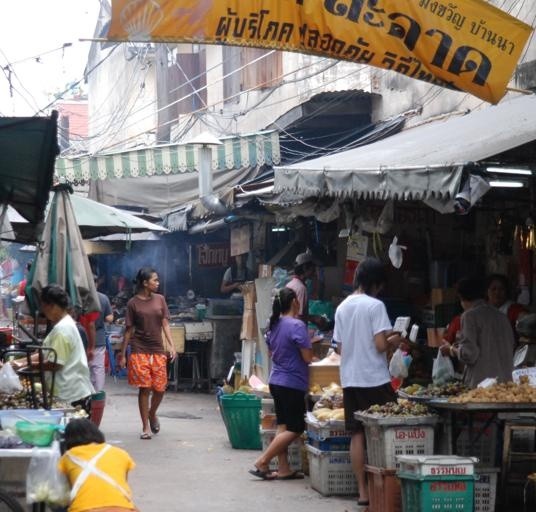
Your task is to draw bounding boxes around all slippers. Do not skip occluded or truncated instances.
[275,471,304,479]
[141,431,150,439]
[248,469,277,479]
[151,419,160,433]
[358,498,369,505]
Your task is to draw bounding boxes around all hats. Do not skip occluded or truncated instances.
[295,253,320,266]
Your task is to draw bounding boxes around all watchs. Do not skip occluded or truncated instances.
[451,346,457,358]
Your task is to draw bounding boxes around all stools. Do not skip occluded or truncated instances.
[178,352,201,393]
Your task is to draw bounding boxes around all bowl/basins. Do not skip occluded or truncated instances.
[1,409,63,428]
[16,421,56,446]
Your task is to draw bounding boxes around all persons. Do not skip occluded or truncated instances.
[79,306,96,363]
[121,267,177,438]
[0,259,34,327]
[10,285,95,418]
[220,252,256,296]
[332,258,403,506]
[249,287,314,479]
[440,282,514,390]
[284,253,327,330]
[481,275,529,328]
[56,418,140,512]
[85,274,113,394]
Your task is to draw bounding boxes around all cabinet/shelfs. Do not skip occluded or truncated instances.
[0,439,60,511]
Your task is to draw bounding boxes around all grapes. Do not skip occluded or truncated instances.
[432,380,472,398]
[357,401,439,418]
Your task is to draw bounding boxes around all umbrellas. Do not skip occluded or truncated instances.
[0,181,171,255]
[26,176,103,334]
[105,328,120,383]
[18,239,127,256]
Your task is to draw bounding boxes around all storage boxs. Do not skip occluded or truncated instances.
[474,468,500,512]
[395,454,479,475]
[364,422,435,468]
[434,419,498,468]
[260,398,276,413]
[260,426,303,473]
[161,326,185,353]
[397,474,479,512]
[306,431,354,452]
[305,420,347,436]
[260,412,278,430]
[361,464,403,512]
[307,365,341,392]
[308,444,360,496]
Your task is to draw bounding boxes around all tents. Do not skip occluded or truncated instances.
[273,91,536,222]
